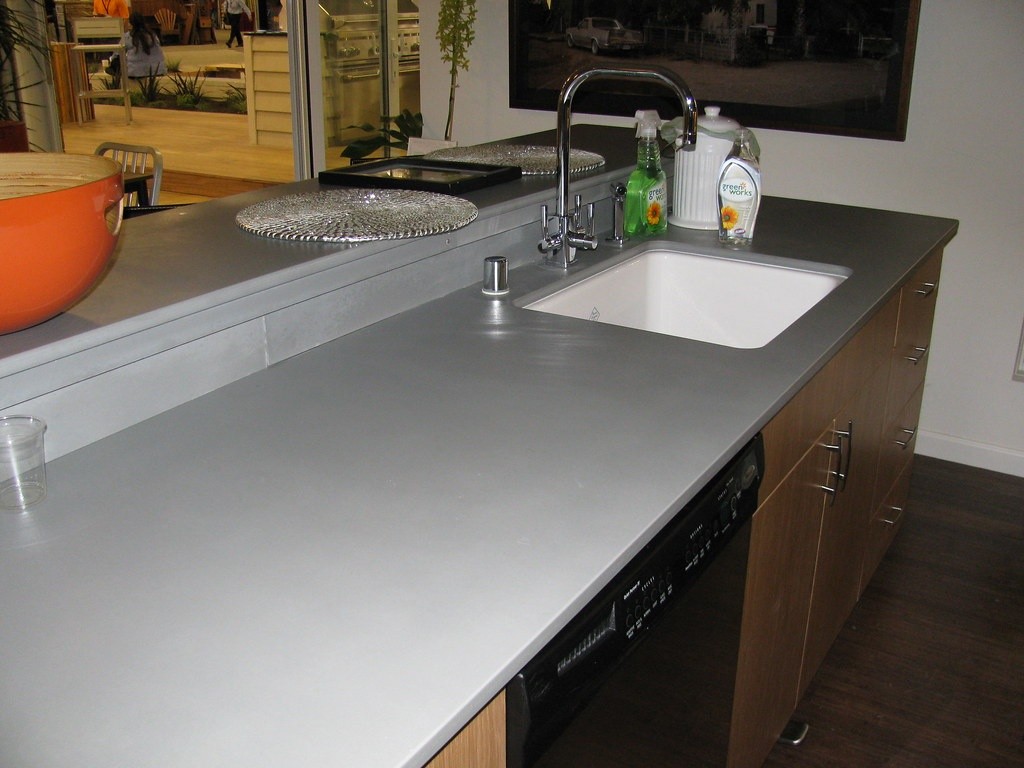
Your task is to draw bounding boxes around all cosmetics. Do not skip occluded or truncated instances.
[623,109,668,239]
[715,125,761,247]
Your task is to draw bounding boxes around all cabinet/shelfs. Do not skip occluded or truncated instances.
[727,248,945,768]
[243,30,294,147]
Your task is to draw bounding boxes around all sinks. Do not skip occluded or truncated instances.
[511,238,856,351]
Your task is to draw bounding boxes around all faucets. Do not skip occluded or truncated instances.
[538,62,698,262]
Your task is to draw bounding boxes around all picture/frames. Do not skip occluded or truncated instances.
[508,0,922,142]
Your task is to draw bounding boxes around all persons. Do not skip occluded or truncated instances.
[106,12,167,77]
[224,0,253,48]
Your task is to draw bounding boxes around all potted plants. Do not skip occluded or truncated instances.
[0,5,62,153]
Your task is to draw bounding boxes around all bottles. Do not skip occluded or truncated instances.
[716,126,762,250]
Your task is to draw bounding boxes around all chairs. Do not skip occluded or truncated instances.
[93,141,163,205]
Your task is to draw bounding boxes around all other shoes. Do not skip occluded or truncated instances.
[235,44,243,47]
[226,42,231,48]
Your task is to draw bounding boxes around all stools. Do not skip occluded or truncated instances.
[72,16,133,125]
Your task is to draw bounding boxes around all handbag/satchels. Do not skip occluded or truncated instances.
[223,1,230,25]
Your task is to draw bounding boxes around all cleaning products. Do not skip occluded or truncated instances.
[715,127,763,252]
[622,109,669,238]
